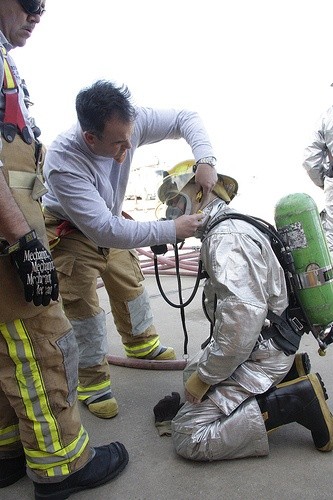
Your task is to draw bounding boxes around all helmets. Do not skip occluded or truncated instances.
[158,158,238,205]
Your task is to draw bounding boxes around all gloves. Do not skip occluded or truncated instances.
[7,228,61,308]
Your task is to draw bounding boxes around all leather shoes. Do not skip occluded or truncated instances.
[0,443,27,490]
[33,440,130,497]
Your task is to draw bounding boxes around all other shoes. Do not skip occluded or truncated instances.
[86,391,120,420]
[154,345,177,362]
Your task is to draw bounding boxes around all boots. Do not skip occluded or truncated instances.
[278,353,313,385]
[253,372,333,453]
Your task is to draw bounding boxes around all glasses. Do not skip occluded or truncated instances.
[14,0,46,16]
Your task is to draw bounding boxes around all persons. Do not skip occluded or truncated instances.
[41,80,332,461]
[0,0,130,500]
